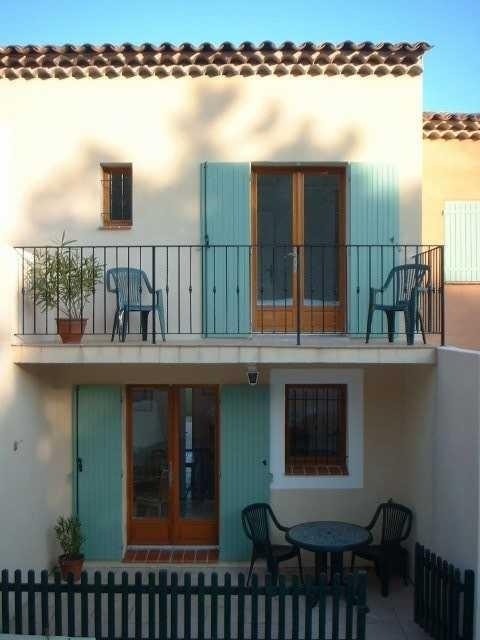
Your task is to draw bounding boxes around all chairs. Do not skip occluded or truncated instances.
[355,499,417,596]
[240,503,305,589]
[107,268,167,342]
[365,262,430,344]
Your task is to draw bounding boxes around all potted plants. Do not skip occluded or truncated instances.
[54,514,87,582]
[24,232,106,344]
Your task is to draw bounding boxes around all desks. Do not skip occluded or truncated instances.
[285,521,374,611]
